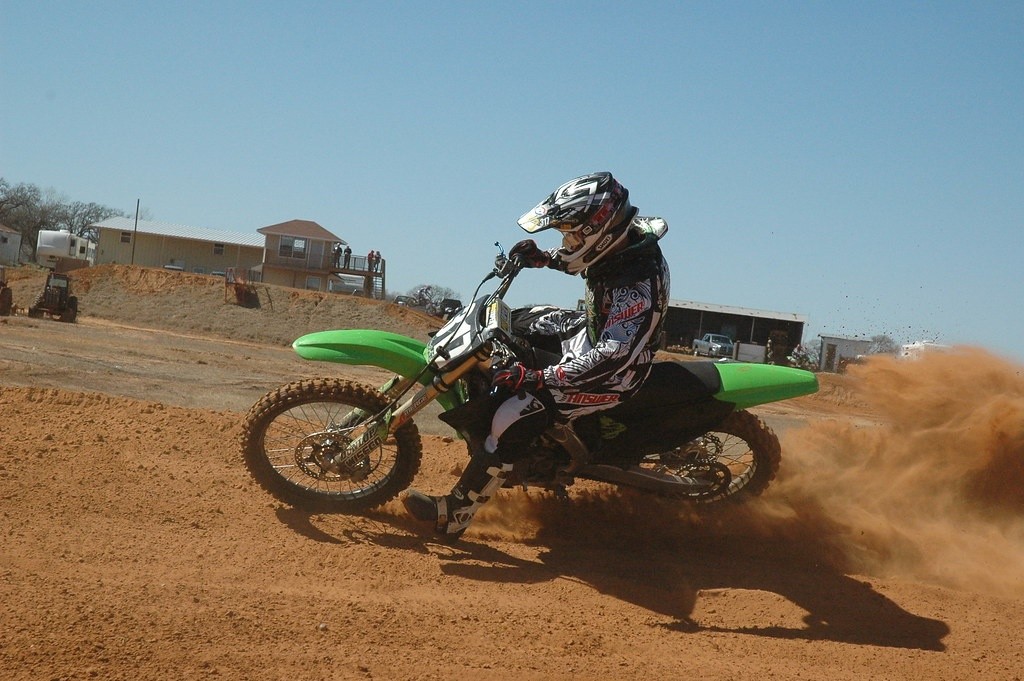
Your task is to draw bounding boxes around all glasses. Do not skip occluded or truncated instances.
[561,232,585,253]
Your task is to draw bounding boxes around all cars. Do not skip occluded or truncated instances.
[394,294,416,308]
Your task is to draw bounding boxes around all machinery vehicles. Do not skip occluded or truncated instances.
[28,271,78,322]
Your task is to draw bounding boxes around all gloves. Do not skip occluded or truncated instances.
[490,365,547,400]
[509,239,552,268]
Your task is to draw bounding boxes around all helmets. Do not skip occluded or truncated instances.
[516,171,639,276]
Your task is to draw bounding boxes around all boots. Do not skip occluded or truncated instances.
[401,451,513,543]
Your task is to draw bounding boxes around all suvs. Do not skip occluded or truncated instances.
[425,297,462,319]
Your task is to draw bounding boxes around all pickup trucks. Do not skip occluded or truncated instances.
[691,334,734,359]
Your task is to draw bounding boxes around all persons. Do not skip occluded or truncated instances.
[766,336,775,365]
[791,344,803,368]
[333,244,342,268]
[367,251,381,273]
[403,173,670,545]
[344,246,351,269]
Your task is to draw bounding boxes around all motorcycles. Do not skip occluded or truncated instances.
[407,292,436,317]
[787,352,814,372]
[239,240,821,515]
